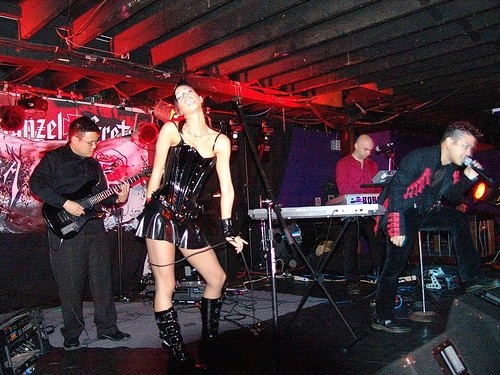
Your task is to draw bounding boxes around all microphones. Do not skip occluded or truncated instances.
[375,142,391,152]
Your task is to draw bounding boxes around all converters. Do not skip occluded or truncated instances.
[47,325,54,334]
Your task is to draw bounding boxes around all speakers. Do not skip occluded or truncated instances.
[446,276,500,330]
[374,315,500,375]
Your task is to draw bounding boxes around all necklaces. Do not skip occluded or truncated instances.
[185,126,208,137]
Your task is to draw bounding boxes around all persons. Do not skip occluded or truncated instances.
[371,120,500,334]
[30,116,131,348]
[335,134,384,299]
[134,81,248,375]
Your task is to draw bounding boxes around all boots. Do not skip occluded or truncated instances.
[197,294,242,364]
[155,305,195,375]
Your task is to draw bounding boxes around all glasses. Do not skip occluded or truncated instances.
[75,136,98,146]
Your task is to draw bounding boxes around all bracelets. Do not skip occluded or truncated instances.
[118,198,126,203]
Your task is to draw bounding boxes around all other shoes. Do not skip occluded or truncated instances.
[464,274,500,292]
[371,317,410,334]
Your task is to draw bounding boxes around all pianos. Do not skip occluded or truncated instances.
[247,203,387,353]
[324,194,411,308]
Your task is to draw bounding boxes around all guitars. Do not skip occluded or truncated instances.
[42,166,154,240]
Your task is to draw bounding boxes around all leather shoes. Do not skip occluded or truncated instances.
[97,329,131,342]
[64,338,80,347]
